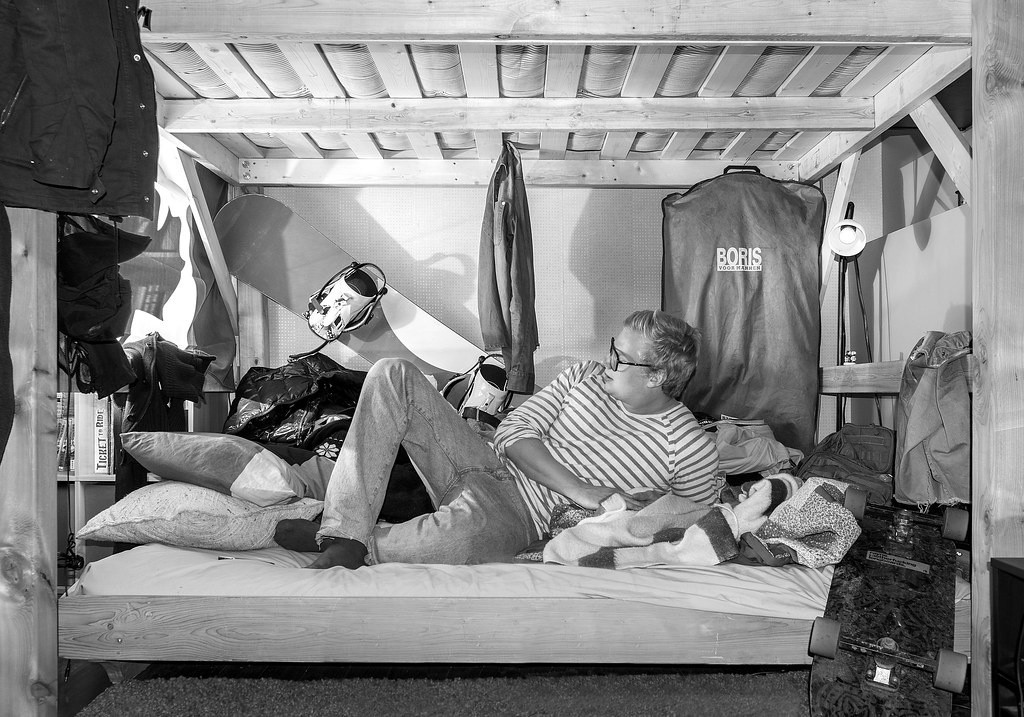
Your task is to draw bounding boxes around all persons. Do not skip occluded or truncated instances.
[273,310,721,570]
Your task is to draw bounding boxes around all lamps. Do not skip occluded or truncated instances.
[826,201,886,431]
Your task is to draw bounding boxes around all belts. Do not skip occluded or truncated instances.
[462,407,501,428]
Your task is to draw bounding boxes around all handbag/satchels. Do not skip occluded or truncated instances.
[57,214,152,342]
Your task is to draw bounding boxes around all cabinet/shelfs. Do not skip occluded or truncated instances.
[57,190,232,591]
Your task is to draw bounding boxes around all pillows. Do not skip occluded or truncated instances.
[119,431,304,507]
[75,480,324,551]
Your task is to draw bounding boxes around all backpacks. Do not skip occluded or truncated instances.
[791,423,894,506]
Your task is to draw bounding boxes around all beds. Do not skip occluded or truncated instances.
[59,359,977,684]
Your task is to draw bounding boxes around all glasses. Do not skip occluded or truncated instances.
[610,337,652,371]
